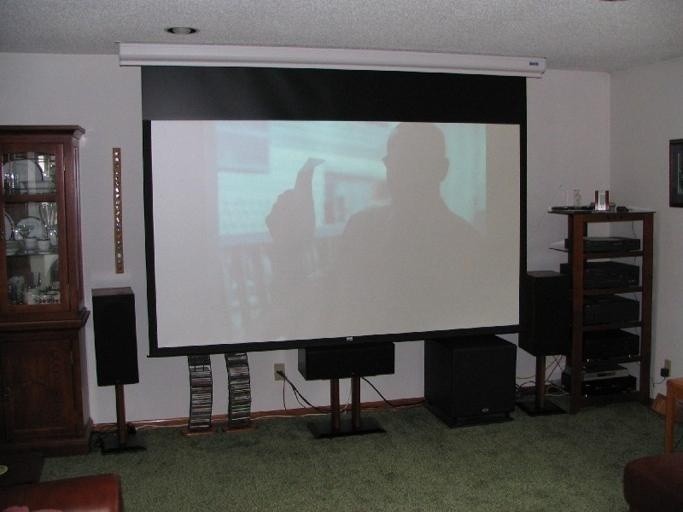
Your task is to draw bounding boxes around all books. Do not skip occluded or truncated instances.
[184,351,253,430]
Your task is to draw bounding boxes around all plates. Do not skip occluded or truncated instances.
[12,217,47,241]
[4,210,14,240]
[2,160,43,194]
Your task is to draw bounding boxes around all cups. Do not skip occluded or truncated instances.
[37,239,50,251]
[22,238,36,250]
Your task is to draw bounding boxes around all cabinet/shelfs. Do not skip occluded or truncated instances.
[0,125,94,457]
[547,208,656,415]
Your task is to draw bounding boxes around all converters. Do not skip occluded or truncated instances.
[661,368,669,377]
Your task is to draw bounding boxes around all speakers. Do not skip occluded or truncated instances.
[92,287,138,386]
[298,340,395,381]
[424,334,517,428]
[518,270,571,358]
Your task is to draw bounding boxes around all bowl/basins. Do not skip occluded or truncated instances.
[4,240,20,256]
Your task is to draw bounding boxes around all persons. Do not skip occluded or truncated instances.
[260,121,509,331]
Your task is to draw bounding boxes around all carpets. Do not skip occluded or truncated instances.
[37,401,680,510]
